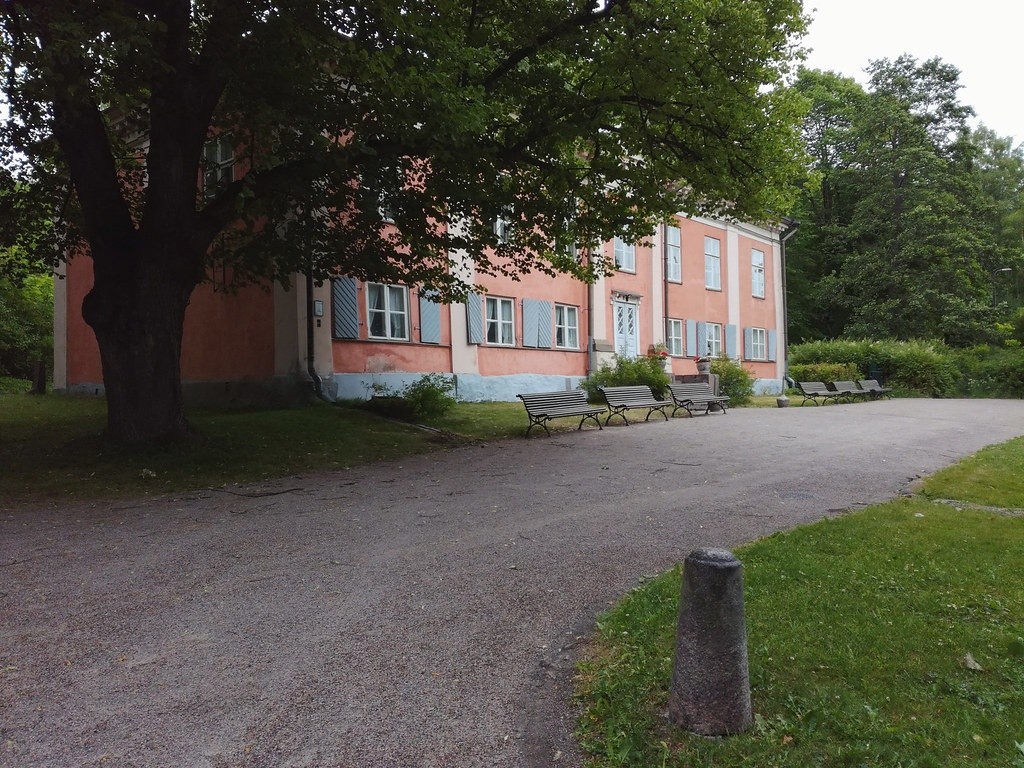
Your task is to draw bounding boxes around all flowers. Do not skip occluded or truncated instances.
[648,342,670,359]
[692,353,711,364]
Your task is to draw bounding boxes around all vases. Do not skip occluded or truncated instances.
[657,357,667,369]
[697,362,711,375]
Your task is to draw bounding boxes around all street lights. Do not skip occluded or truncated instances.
[994,267,1013,306]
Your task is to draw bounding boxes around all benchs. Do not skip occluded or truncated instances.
[665,382,730,418]
[516,389,608,440]
[798,380,892,407]
[596,384,673,427]
